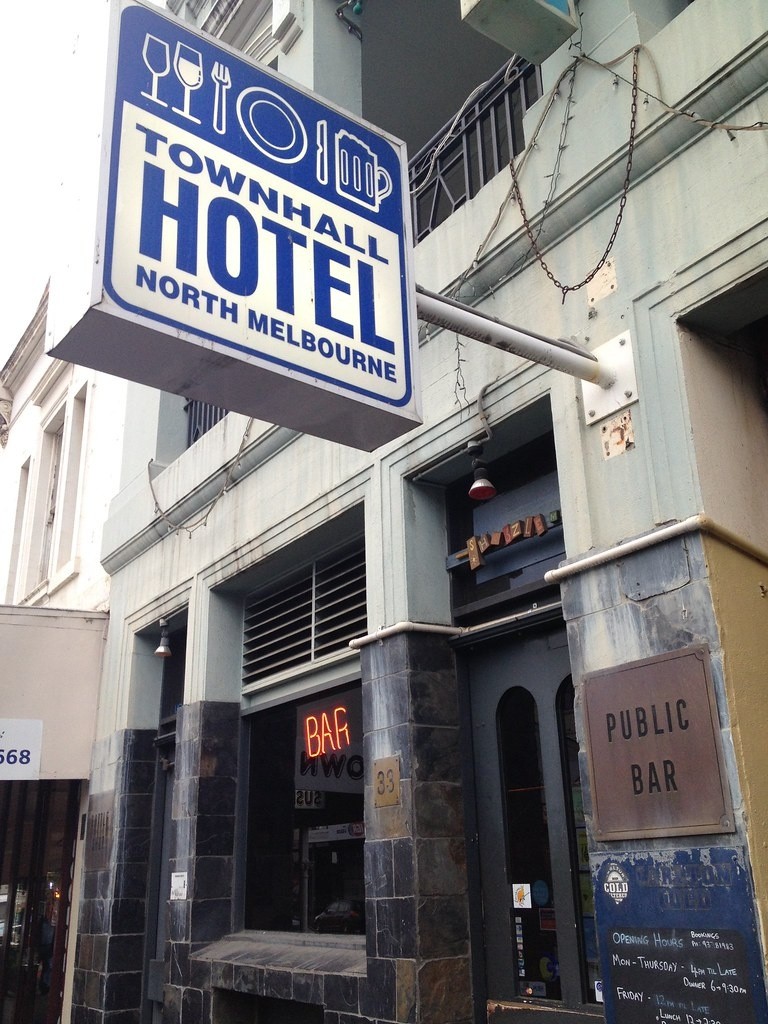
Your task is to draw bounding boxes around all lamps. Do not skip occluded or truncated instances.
[465,440,498,500]
[153,618,171,657]
